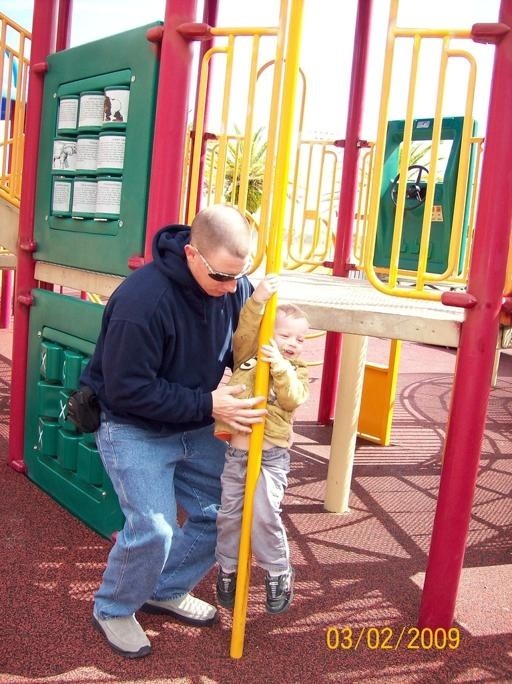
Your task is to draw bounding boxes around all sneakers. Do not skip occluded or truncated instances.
[216,567,236,609]
[264,565,295,615]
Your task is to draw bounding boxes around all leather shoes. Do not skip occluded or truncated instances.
[139,593,220,626]
[91,611,151,659]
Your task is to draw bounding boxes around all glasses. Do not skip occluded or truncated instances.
[194,250,251,282]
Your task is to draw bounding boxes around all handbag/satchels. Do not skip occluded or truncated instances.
[64,391,101,433]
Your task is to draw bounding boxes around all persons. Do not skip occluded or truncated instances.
[77,205,269,661]
[216,272,311,613]
